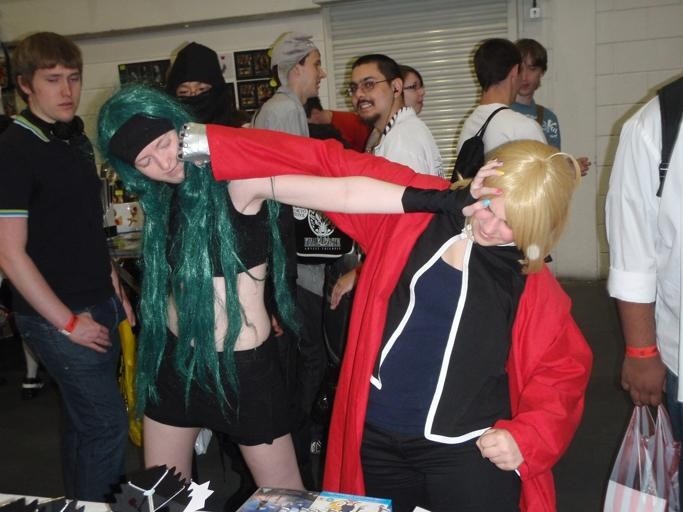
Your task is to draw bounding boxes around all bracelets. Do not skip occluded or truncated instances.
[625,345,658,360]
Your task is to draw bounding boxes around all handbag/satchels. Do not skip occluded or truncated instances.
[451,107,515,185]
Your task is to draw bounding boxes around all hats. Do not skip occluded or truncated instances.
[270,35,318,87]
[167,43,224,93]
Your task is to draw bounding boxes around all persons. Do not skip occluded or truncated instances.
[164,43,242,128]
[605,76,682,511]
[511,37,561,153]
[310,65,426,151]
[1,31,136,504]
[178,121,593,510]
[452,37,591,183]
[97,84,505,490]
[331,53,442,309]
[251,33,327,420]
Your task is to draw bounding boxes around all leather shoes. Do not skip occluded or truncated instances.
[20,378,45,399]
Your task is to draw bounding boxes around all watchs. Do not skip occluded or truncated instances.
[59,315,79,335]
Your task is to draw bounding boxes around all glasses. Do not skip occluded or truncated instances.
[345,78,390,97]
[402,82,424,91]
